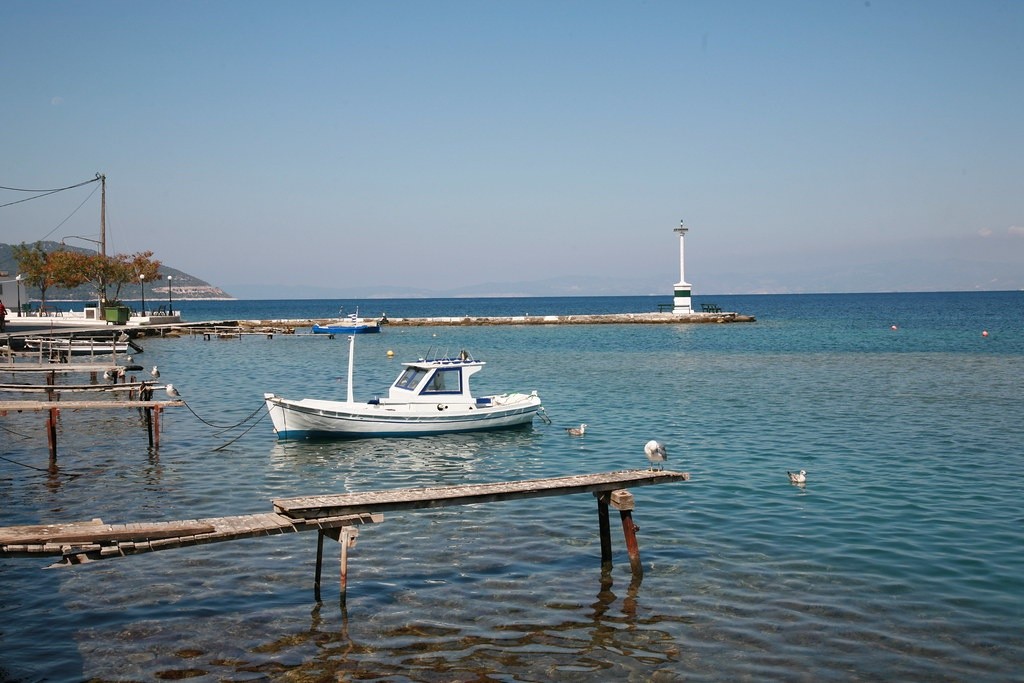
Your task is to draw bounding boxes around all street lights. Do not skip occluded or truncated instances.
[166,275,175,316]
[60,234,108,320]
[139,274,147,317]
[16,274,22,317]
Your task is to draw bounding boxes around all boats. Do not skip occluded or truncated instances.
[312,317,382,334]
[263,336,541,444]
[24,336,130,355]
